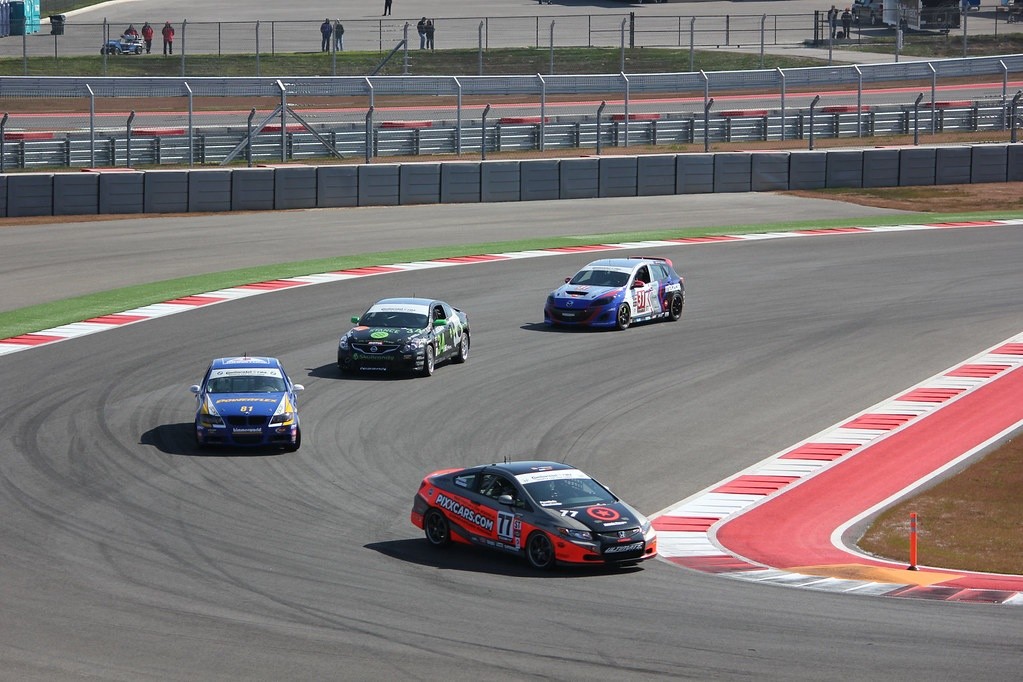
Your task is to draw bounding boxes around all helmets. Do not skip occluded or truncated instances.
[416,315,427,323]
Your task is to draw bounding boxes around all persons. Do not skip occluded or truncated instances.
[500,484,525,508]
[827,5,838,39]
[251,377,279,392]
[162,21,174,54]
[841,7,853,39]
[544,480,563,498]
[333,18,344,51]
[382,0,392,16]
[142,21,153,54]
[124,24,139,39]
[417,17,435,50]
[321,18,332,52]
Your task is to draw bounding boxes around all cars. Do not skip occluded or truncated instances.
[851,0,884,26]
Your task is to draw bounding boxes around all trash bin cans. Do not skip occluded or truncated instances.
[48,14,65,35]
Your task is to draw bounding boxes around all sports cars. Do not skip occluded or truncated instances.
[543,254,687,331]
[337,296,472,378]
[410,460,658,574]
[191,355,306,453]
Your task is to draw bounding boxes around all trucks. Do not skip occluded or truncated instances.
[879,0,963,32]
